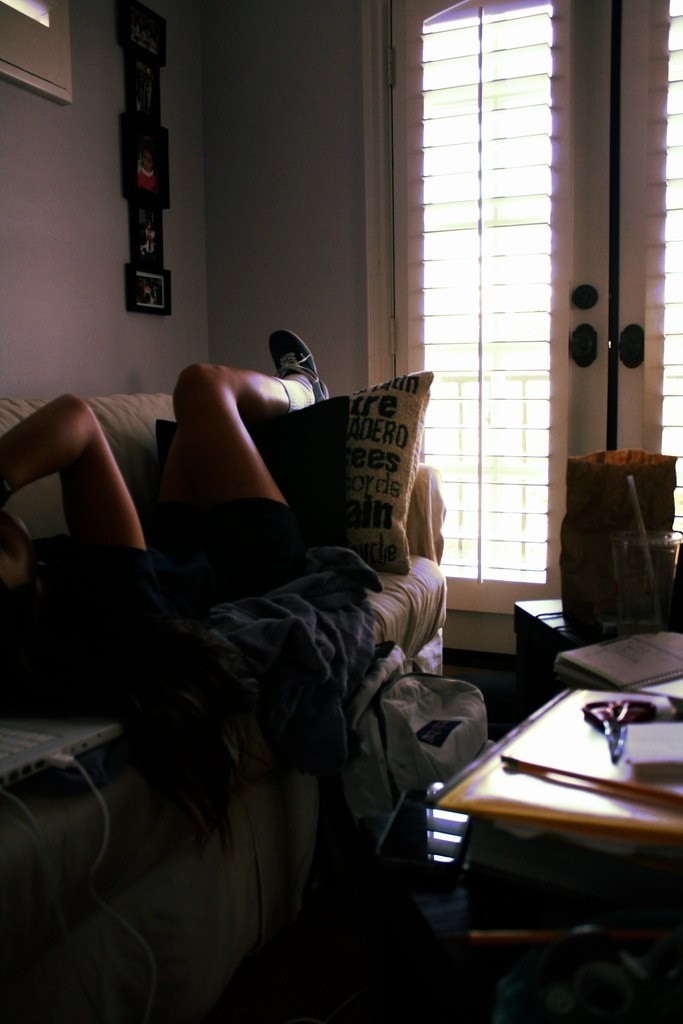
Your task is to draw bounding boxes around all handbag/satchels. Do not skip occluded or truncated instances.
[560,451,678,634]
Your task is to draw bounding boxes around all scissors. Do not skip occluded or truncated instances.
[582,698,657,765]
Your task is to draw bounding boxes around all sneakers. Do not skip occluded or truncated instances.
[268,328,329,405]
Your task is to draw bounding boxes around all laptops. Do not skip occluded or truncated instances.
[0,716,125,790]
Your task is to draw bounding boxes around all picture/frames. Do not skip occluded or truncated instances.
[114,0,173,316]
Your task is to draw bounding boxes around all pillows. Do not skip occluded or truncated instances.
[349,372,434,578]
[153,394,352,547]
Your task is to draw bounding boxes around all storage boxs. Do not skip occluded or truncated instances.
[514,598,583,682]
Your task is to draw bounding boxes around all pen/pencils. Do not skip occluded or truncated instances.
[500,753,683,809]
[468,928,668,945]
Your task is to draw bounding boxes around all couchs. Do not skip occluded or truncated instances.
[0,369,449,1024]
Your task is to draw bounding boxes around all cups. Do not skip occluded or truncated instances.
[611,529,683,636]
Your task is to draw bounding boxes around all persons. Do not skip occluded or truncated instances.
[0,329,329,717]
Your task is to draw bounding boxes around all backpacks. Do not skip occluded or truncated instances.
[337,641,495,865]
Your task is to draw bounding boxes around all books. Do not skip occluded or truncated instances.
[553,632,683,693]
